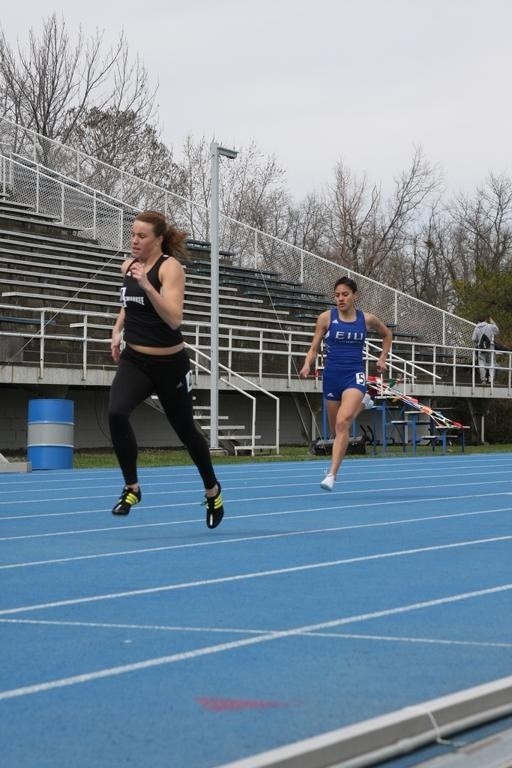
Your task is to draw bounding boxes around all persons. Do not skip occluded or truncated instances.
[298,276,393,491]
[107,210,223,528]
[471,315,500,387]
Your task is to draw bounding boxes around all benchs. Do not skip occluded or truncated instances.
[0,175,481,381]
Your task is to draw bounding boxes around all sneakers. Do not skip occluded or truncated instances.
[321,473,335,490]
[112,487,141,515]
[205,481,223,528]
[361,393,374,409]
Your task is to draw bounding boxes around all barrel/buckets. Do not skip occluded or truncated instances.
[26,399,75,470]
